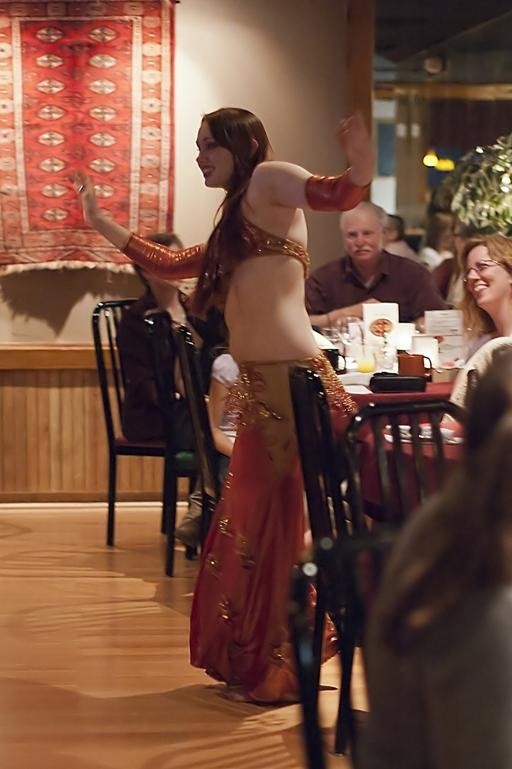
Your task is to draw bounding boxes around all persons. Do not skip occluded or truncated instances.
[57,104,376,710]
[116,233,231,549]
[207,349,244,487]
[305,200,510,769]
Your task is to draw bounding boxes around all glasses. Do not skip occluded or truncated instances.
[462,257,506,285]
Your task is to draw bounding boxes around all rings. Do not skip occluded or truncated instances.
[75,184,88,191]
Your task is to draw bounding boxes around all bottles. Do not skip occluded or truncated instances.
[321,327,396,373]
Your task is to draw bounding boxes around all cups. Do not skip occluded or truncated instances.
[396,354,432,380]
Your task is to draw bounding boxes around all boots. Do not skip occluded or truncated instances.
[171,469,216,549]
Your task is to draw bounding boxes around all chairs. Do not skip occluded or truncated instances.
[91,297,475,767]
